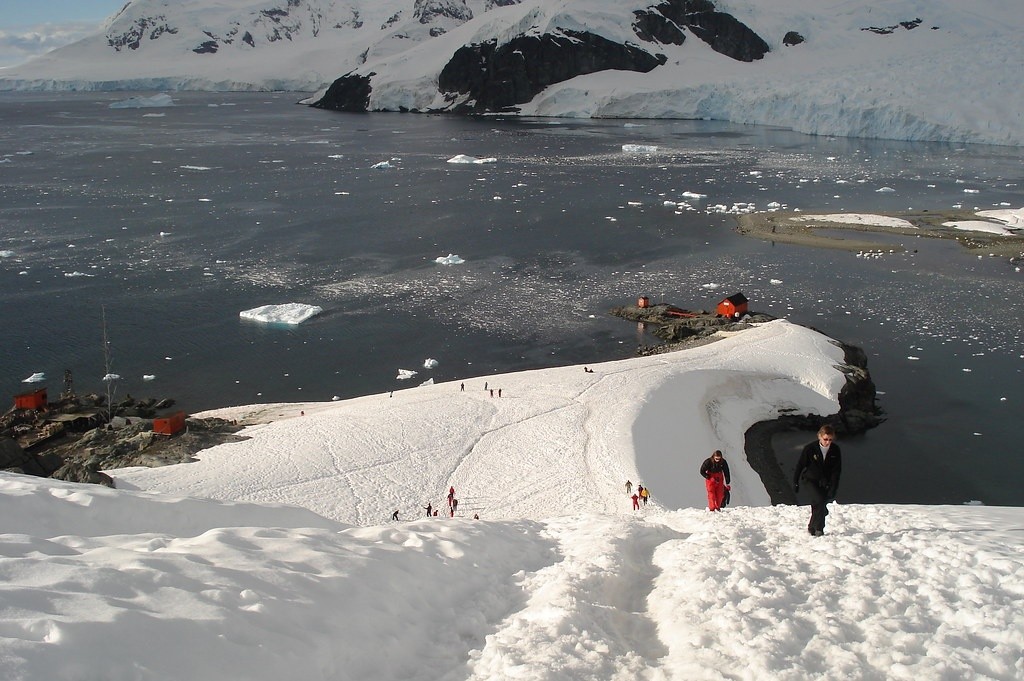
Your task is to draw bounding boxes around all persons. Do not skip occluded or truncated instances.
[490,389,494,397]
[461,382,464,391]
[423,503,431,517]
[700,450,731,511]
[631,494,640,511]
[499,389,501,397]
[793,425,842,537]
[625,480,632,493]
[474,514,479,519]
[450,486,455,500]
[433,510,438,516]
[720,485,731,508]
[584,366,588,372]
[451,507,454,517]
[485,382,488,389]
[447,494,453,506]
[641,487,650,506]
[587,369,593,373]
[390,391,393,397]
[638,485,643,500]
[392,510,399,521]
[452,499,458,511]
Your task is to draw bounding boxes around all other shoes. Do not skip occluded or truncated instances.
[808,527,824,537]
[716,508,721,511]
[710,509,715,511]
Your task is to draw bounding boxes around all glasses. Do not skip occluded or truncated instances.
[714,458,722,460]
[821,435,833,441]
